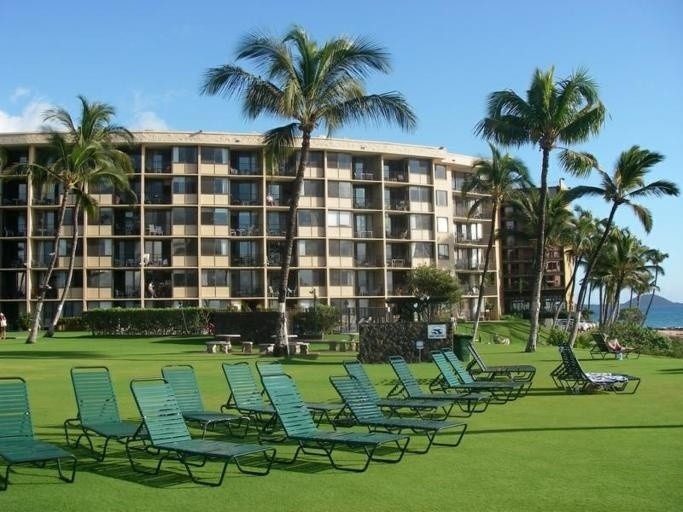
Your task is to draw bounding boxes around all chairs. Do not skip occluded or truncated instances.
[255,362,347,432]
[0,376,78,491]
[431,339,536,412]
[217,362,285,443]
[549,332,644,396]
[343,359,454,433]
[64,366,160,461]
[259,373,410,473]
[126,378,276,487]
[161,364,251,441]
[386,356,492,422]
[329,375,467,454]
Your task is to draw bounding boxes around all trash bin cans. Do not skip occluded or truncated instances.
[454,334,473,362]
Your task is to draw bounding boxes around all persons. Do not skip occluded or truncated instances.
[0,312,8,339]
[147,281,158,297]
[602,333,626,353]
[266,192,275,206]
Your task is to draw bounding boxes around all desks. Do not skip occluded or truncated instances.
[215,334,241,353]
[269,334,298,339]
[341,332,358,352]
